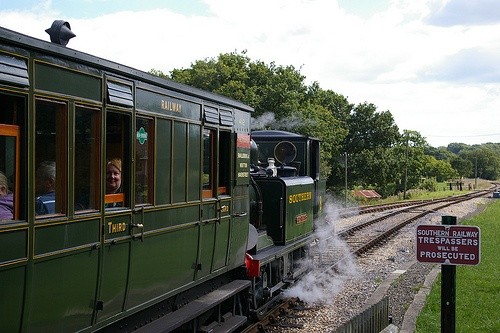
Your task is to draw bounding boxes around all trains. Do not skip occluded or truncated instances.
[0,19,334,333]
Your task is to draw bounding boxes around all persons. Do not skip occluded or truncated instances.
[0,174,14,220]
[104,159,121,207]
[35,160,56,215]
